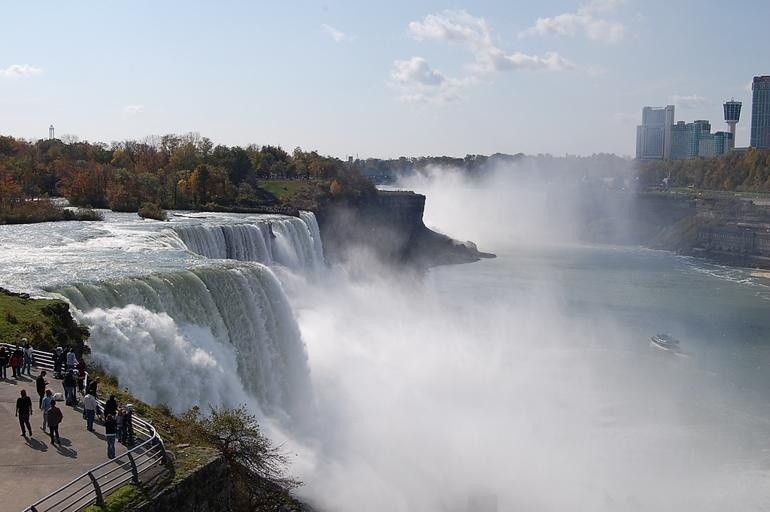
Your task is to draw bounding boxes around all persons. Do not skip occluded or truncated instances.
[0,343,34,379]
[16,390,33,437]
[40,389,56,432]
[115,399,128,442]
[45,399,63,444]
[83,389,96,431]
[36,370,48,409]
[104,413,117,460]
[87,375,99,395]
[51,344,86,406]
[123,398,134,446]
[104,393,117,420]
